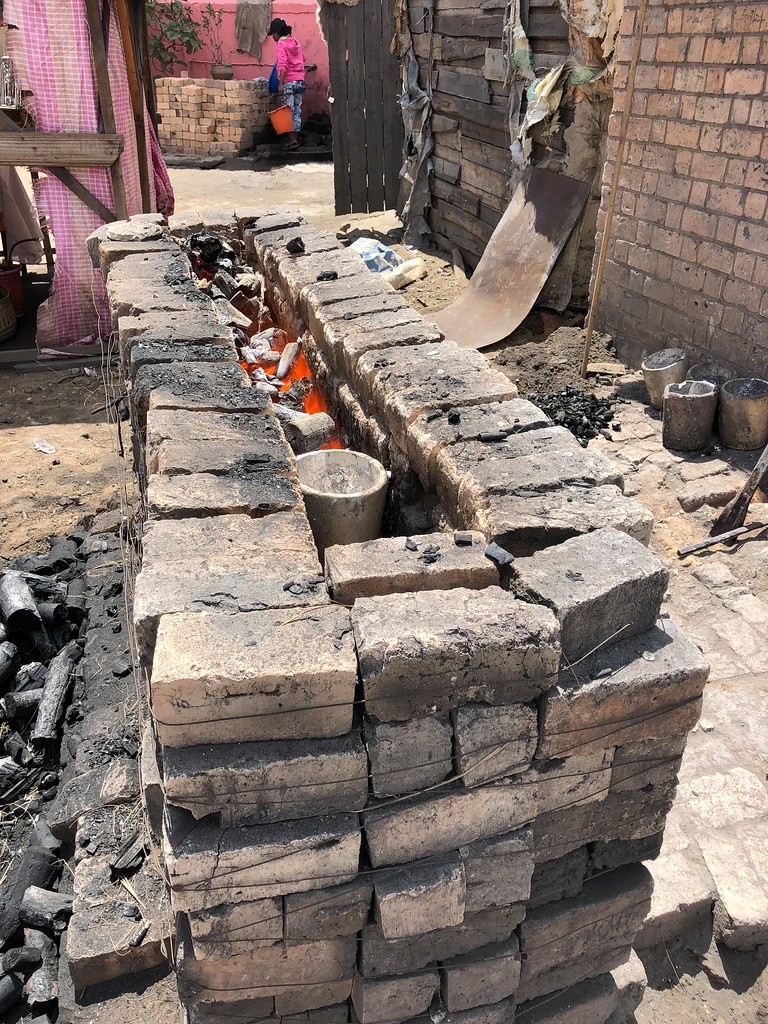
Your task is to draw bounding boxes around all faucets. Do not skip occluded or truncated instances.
[307,67,314,73]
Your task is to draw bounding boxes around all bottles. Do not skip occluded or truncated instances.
[0,56,20,109]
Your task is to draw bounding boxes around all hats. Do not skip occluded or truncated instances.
[267,18,287,36]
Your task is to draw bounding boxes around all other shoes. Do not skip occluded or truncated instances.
[282,140,299,151]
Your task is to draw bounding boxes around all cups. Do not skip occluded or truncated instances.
[180,71,188,78]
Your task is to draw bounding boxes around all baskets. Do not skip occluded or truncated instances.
[0,284,17,340]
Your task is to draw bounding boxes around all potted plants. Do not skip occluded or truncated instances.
[201,2,233,80]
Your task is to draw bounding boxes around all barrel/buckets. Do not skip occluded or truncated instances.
[267,93,294,135]
[662,380,719,450]
[720,378,768,449]
[687,362,737,389]
[641,347,687,410]
[296,449,392,560]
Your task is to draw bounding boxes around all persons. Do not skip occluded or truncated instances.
[267,17,307,151]
